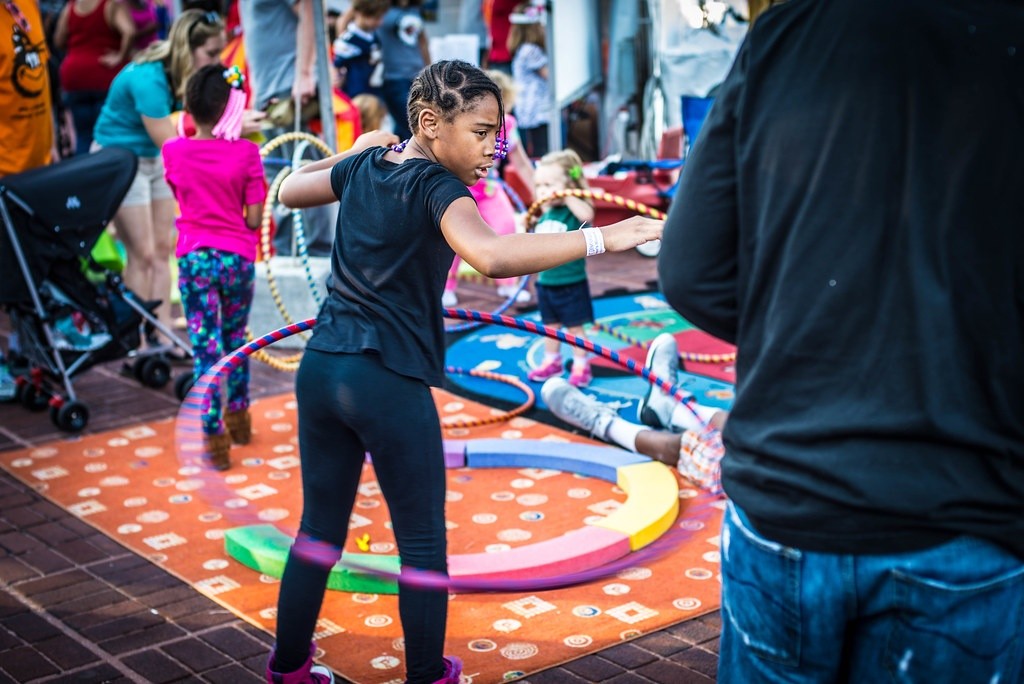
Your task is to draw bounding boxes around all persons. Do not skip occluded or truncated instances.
[658,0,1024,684]
[0,0,433,471]
[542,334,730,501]
[528,148,596,389]
[266,61,665,684]
[479,1,550,157]
[442,69,532,307]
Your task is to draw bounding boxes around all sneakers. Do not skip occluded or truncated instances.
[636,332,697,433]
[541,377,632,441]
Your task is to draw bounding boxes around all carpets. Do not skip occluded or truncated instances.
[0,386,724,684]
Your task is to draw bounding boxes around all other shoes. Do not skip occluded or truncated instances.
[267,643,335,684]
[222,407,250,444]
[432,656,461,684]
[498,284,532,304]
[570,360,593,387]
[200,435,230,470]
[441,290,457,308]
[527,351,563,381]
[164,349,192,366]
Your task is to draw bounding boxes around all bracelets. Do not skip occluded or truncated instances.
[579,227,606,259]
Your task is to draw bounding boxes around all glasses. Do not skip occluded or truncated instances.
[189,11,220,37]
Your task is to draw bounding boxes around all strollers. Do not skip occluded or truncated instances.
[0,147,196,435]
